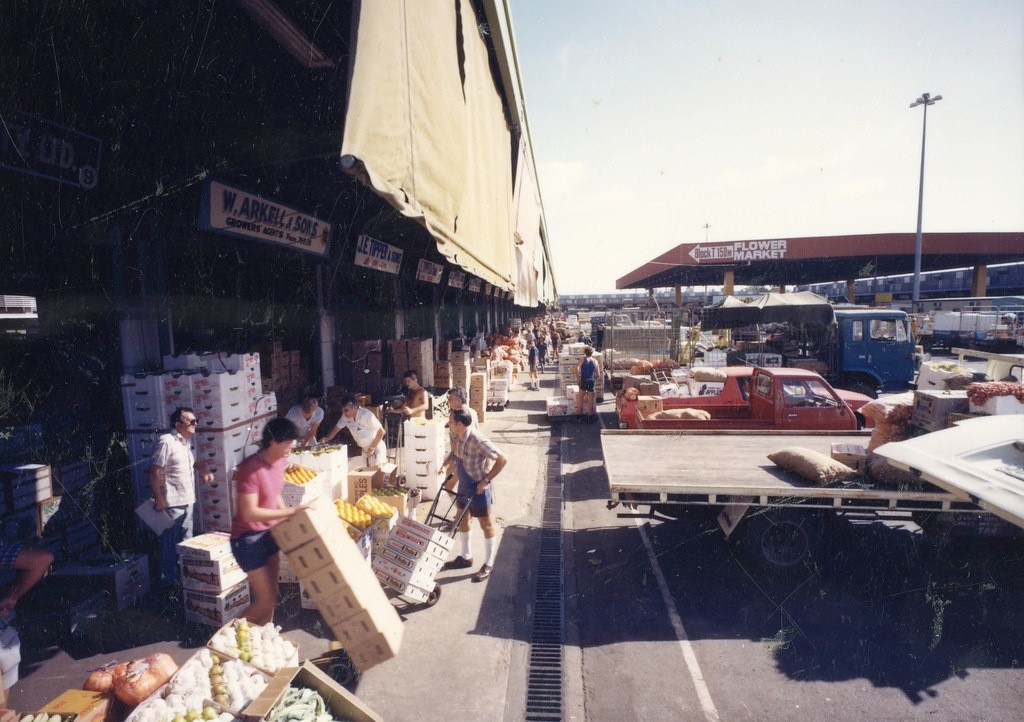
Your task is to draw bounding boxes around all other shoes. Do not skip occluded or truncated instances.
[473,563,493,581]
[444,556,473,569]
[527,387,539,390]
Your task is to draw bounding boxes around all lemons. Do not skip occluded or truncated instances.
[284,467,319,484]
[335,495,396,541]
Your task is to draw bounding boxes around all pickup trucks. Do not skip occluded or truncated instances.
[616,367,886,430]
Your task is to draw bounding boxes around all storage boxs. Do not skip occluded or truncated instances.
[569,400,590,415]
[831,443,867,470]
[11,318,521,722]
[641,412,653,418]
[920,362,956,387]
[735,341,782,368]
[567,385,580,400]
[917,374,950,390]
[969,394,1024,415]
[573,388,596,402]
[911,389,969,431]
[558,353,604,398]
[577,312,613,351]
[990,324,1007,330]
[672,369,690,383]
[690,380,724,397]
[638,395,663,412]
[933,312,1002,331]
[569,345,589,354]
[547,400,569,416]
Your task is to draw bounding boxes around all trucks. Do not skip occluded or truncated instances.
[726,309,923,398]
[600,347,1024,567]
[909,306,1019,354]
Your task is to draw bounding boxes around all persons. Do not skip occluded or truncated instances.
[574,347,600,423]
[782,335,796,355]
[229,417,318,626]
[0,542,55,709]
[461,317,561,391]
[559,328,571,341]
[149,407,214,618]
[316,393,388,466]
[437,386,479,529]
[911,317,916,339]
[444,408,507,581]
[385,371,429,420]
[578,330,592,346]
[284,384,324,451]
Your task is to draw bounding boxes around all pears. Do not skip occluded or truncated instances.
[372,487,409,497]
[171,623,258,722]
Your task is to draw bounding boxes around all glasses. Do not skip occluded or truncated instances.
[181,420,199,426]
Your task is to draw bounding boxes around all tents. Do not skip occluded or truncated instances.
[699,291,837,331]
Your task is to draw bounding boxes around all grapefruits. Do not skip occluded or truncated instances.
[85,653,178,709]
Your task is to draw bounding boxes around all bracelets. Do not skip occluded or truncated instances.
[369,446,375,450]
[484,478,490,484]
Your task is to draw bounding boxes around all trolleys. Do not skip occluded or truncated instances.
[384,413,410,472]
[317,609,357,685]
[393,473,491,607]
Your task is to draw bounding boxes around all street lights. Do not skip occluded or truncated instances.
[909,92,943,313]
